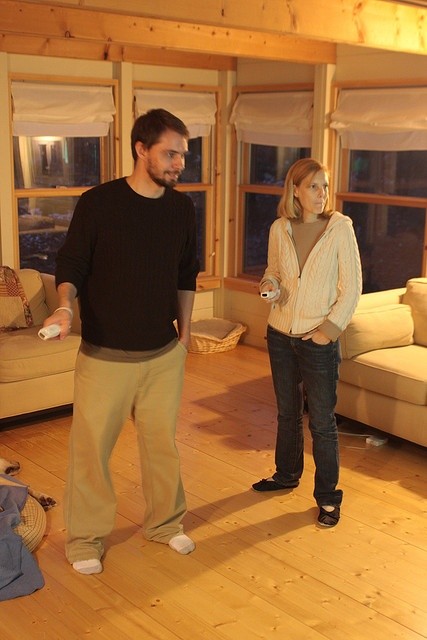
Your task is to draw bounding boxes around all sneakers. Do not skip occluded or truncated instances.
[317,502,340,527]
[252,477,299,491]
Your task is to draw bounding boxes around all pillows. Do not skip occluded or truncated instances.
[1,265,52,333]
[402,282,427,348]
[338,301,415,360]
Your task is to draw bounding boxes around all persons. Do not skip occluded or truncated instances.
[251,159,364,527]
[41,108,199,574]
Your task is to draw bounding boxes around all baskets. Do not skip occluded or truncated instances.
[189,316,247,354]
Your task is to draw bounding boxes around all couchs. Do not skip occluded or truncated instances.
[0,267,85,422]
[336,276,427,449]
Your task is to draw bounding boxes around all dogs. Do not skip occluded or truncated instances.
[0,456,57,512]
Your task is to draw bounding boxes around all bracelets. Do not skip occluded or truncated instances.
[52,306,73,320]
